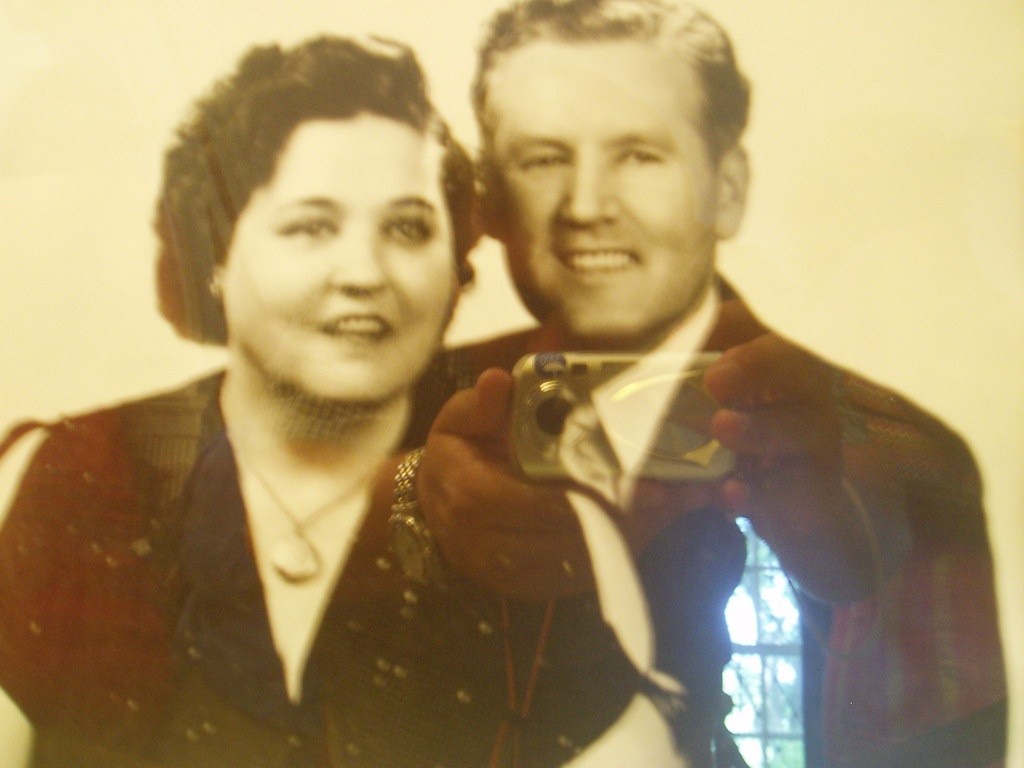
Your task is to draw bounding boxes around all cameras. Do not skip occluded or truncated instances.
[509,351,737,481]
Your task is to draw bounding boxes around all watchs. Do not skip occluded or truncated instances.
[383,446,441,588]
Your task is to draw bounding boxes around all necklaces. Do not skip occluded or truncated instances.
[228,449,378,581]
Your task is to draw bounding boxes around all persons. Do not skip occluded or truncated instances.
[0,1,1009,766]
